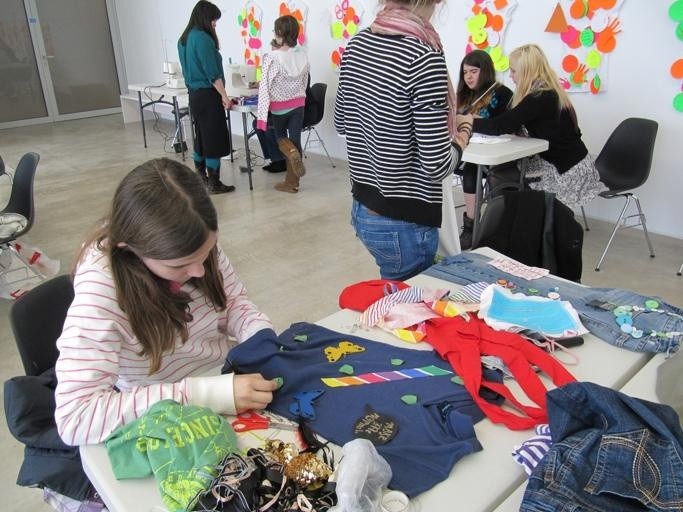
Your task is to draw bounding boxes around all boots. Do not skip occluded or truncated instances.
[194,161,235,193]
[459,211,474,250]
[274,137,306,193]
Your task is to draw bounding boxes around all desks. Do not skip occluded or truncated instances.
[187,85,260,191]
[78,245,654,511]
[460,133,551,250]
[127,80,187,163]
[493,347,683,511]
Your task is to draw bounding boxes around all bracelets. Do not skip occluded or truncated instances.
[455,121,474,138]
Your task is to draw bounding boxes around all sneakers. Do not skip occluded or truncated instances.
[262,159,287,172]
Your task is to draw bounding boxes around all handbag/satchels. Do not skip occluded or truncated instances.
[304,95,322,124]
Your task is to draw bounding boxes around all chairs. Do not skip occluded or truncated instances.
[1,151,48,284]
[301,82,335,169]
[8,273,77,376]
[580,117,659,271]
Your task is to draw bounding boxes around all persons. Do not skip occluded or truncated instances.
[452,50,515,249]
[334,0,474,284]
[248,39,317,172]
[177,0,236,191]
[471,45,604,207]
[256,15,310,192]
[53,158,284,447]
